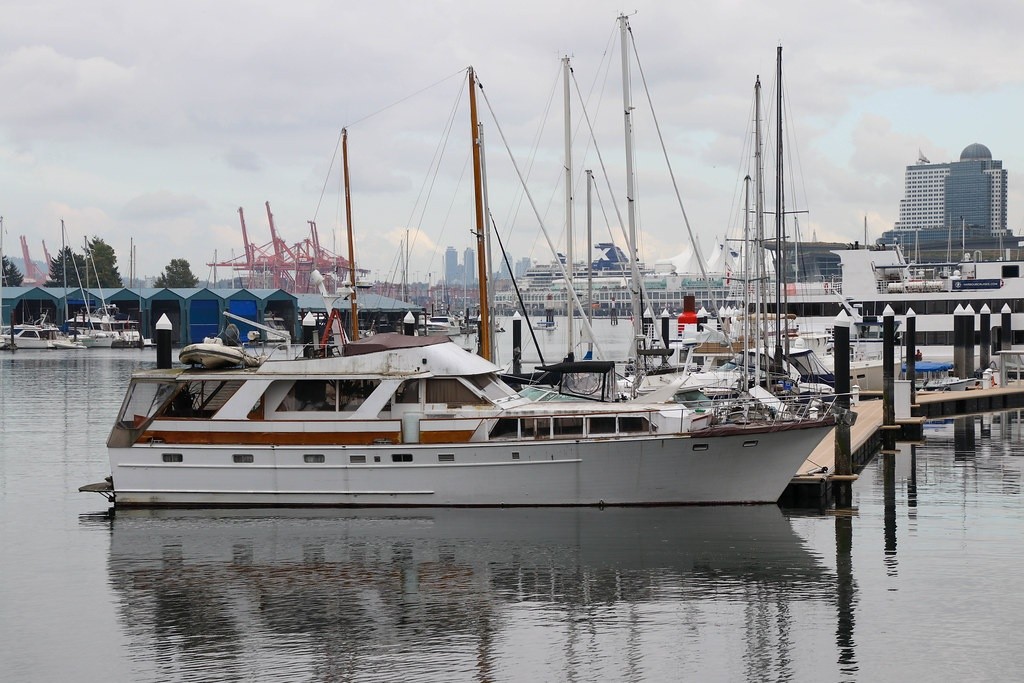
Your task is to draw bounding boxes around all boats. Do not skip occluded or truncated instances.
[178,337,259,369]
[77,334,857,509]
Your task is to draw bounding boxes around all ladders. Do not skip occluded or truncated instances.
[319,307,347,355]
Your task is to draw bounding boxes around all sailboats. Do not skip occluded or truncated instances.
[0,216,157,350]
[289,17,1024,408]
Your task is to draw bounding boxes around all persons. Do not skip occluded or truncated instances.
[915,349,922,362]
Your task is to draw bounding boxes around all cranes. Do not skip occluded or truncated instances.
[205,202,372,295]
